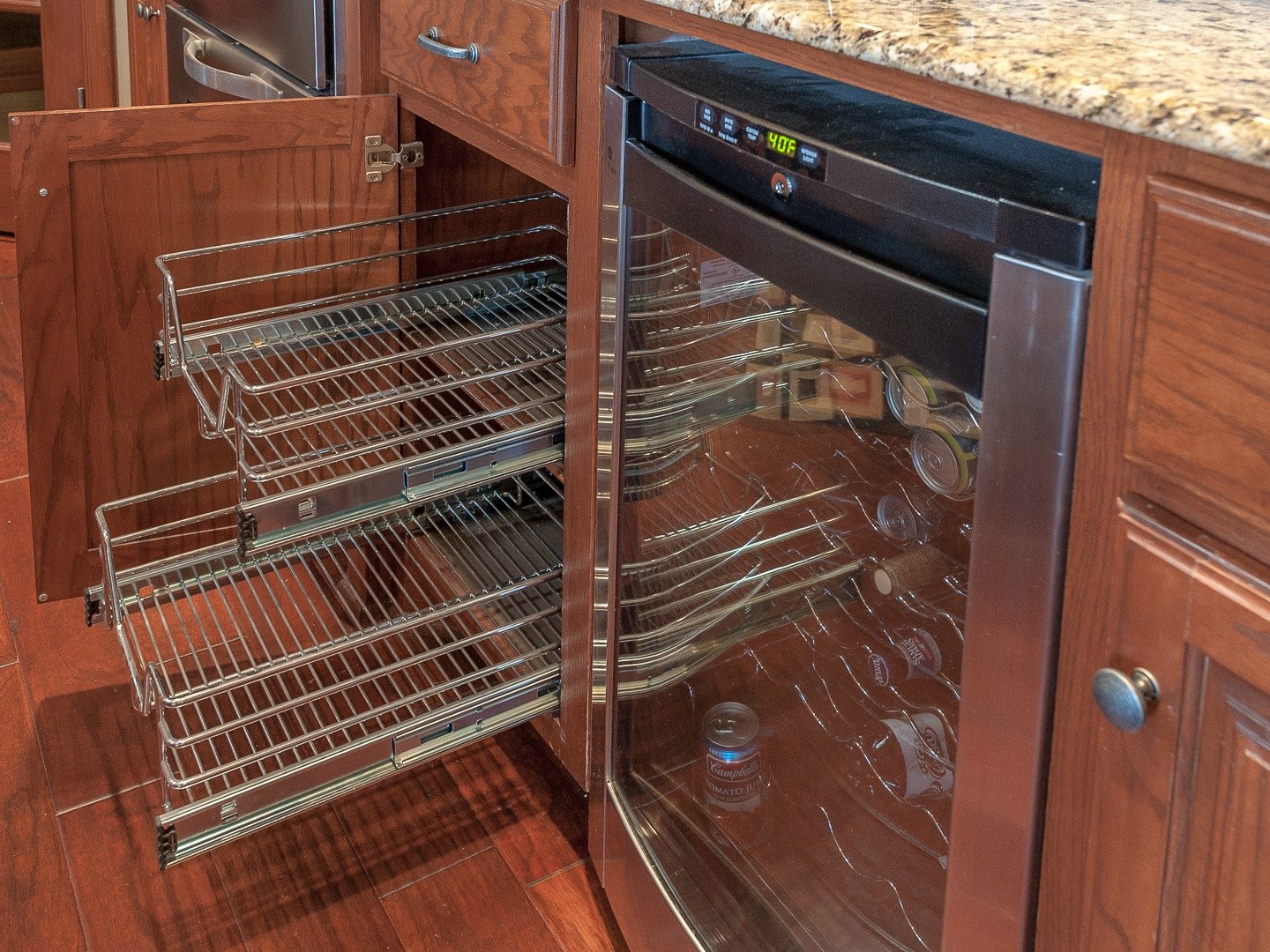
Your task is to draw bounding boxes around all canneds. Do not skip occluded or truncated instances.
[700,703,765,846]
[874,363,985,546]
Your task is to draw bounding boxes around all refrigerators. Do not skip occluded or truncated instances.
[585,39,1103,951]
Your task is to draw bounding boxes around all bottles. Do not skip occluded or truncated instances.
[866,617,964,685]
[871,525,970,599]
[851,702,955,803]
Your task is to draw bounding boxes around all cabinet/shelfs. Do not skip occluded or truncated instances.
[0,0,1270,952]
